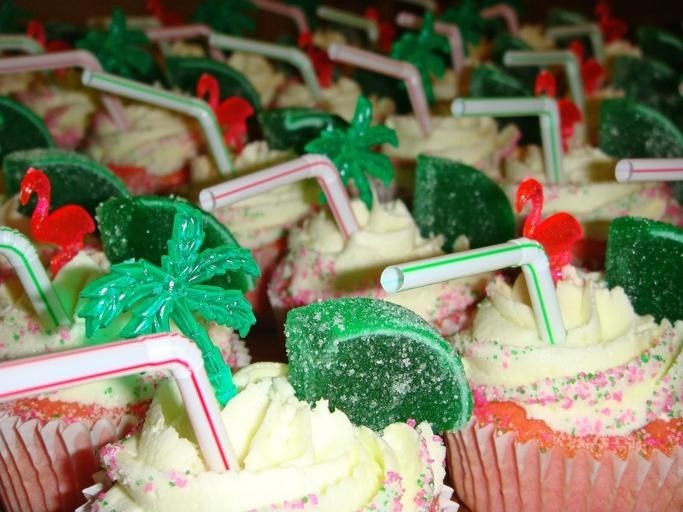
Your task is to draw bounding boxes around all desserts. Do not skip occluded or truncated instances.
[0,0,682,511]
